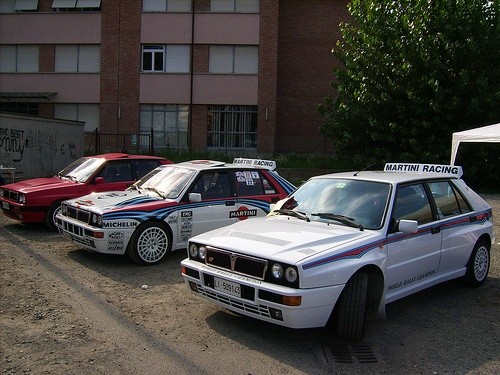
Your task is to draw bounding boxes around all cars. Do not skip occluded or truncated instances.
[0,152,177,232]
[180,163,495,345]
[54,158,299,265]
